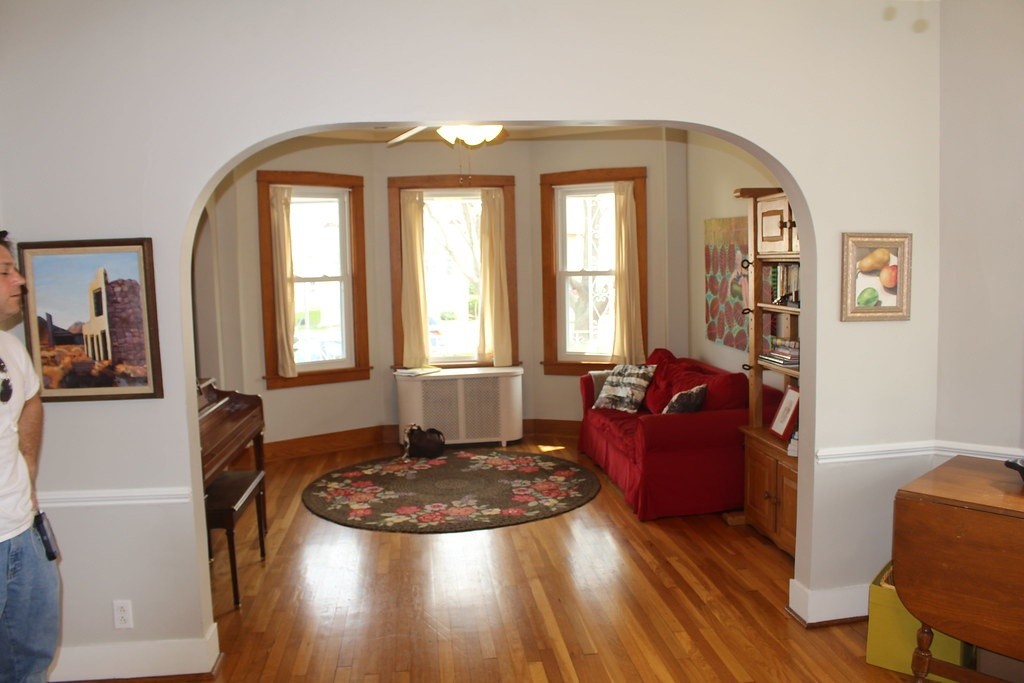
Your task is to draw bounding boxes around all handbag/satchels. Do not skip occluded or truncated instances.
[401,423,446,464]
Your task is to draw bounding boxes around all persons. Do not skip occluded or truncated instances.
[0,231,62,682]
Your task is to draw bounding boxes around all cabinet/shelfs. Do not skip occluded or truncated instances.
[733,187,801,560]
[396,366,524,459]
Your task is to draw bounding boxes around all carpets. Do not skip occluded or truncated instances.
[300,452,601,536]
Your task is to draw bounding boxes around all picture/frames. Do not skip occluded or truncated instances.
[841,232,911,321]
[16,237,166,404]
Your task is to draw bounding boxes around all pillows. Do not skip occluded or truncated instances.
[643,356,747,414]
[592,364,657,414]
[662,384,707,413]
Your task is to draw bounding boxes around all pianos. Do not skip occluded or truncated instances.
[196,377,267,534]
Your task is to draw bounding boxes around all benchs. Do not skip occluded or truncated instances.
[202,468,268,608]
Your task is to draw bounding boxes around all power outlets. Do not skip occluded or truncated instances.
[112,599,132,628]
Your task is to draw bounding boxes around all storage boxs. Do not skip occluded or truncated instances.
[866,559,975,682]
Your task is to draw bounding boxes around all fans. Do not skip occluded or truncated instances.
[384,126,427,144]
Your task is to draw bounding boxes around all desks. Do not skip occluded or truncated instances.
[890,454,1024,683]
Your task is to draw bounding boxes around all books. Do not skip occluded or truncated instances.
[787,431,798,457]
[770,263,799,305]
[759,337,800,367]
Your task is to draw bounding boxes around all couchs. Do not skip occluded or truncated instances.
[576,347,785,523]
